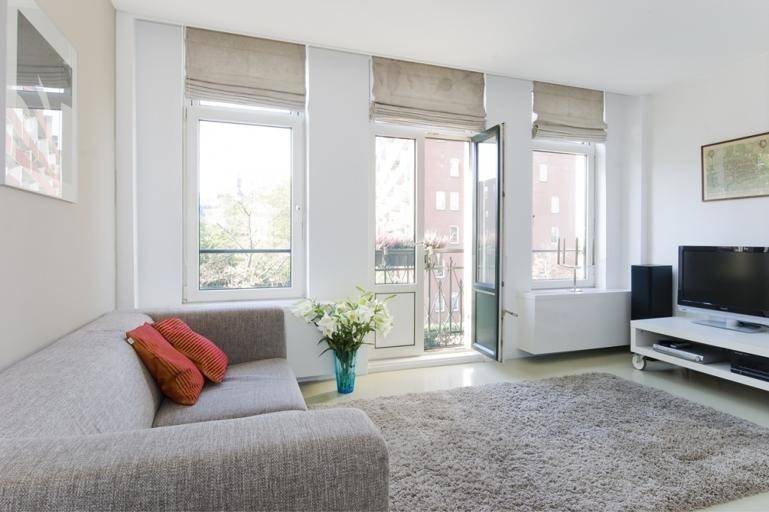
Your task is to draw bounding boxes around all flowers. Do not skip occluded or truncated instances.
[292,285,398,386]
[373,229,455,250]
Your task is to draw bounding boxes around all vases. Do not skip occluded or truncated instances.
[374,250,415,265]
[333,346,356,395]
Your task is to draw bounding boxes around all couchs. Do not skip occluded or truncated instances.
[1,306,389,512]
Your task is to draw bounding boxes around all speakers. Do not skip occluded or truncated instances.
[631,265,672,319]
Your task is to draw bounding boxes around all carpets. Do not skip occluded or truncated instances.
[318,370,768,512]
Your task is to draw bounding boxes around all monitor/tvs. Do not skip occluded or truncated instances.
[677,246,769,333]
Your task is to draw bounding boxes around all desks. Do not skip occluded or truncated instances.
[629,316,768,393]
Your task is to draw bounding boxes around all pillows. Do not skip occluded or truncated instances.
[125,316,231,408]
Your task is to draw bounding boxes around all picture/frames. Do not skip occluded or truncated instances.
[0,0,77,207]
[701,132,769,202]
